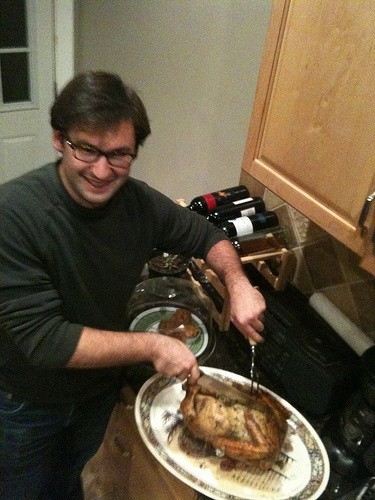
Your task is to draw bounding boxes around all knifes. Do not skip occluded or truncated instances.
[186,369,274,416]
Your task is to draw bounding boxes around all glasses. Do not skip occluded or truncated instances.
[61,132,137,167]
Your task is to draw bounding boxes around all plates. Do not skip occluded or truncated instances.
[134,365,331,500]
[128,307,209,361]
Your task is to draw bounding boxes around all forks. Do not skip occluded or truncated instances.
[246,334,262,395]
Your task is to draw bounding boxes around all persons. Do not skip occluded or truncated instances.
[0,71,265,500]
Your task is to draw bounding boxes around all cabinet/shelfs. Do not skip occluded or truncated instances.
[240,0,375,277]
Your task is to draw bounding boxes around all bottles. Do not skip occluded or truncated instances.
[185,185,293,257]
